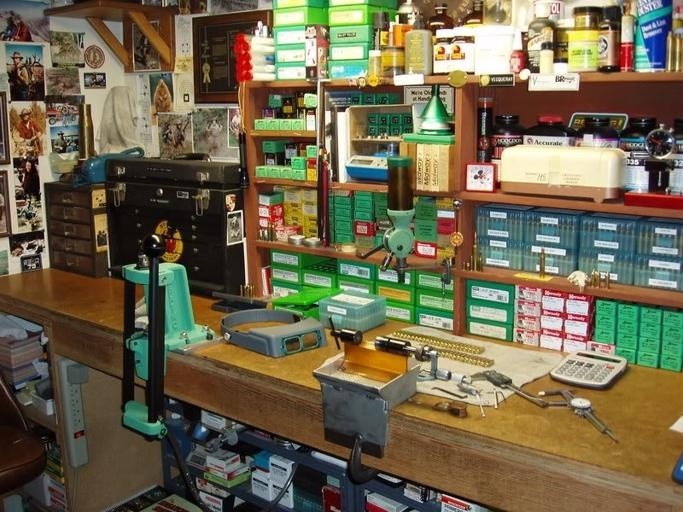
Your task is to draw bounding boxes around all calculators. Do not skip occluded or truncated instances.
[550,351,628,389]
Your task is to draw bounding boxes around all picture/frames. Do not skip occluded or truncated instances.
[0,91,11,164]
[192,8,273,103]
[0,170,12,238]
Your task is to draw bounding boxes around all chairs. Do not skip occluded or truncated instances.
[0,382,49,511]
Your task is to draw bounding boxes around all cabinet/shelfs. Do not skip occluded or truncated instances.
[44,182,111,278]
[239,70,683,372]
[106,158,246,297]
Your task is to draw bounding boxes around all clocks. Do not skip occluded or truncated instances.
[623,124,683,210]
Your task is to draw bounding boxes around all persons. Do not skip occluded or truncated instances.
[1,8,81,207]
[161,107,239,155]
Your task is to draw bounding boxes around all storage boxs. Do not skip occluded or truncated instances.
[268,0,399,80]
[267,249,515,345]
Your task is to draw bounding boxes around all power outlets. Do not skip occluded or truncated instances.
[57,357,91,469]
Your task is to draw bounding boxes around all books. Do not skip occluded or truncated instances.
[0,331,45,408]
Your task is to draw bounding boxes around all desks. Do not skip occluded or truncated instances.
[0,268,683,512]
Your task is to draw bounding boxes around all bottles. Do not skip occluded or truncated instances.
[77,103,96,160]
[489,116,683,199]
